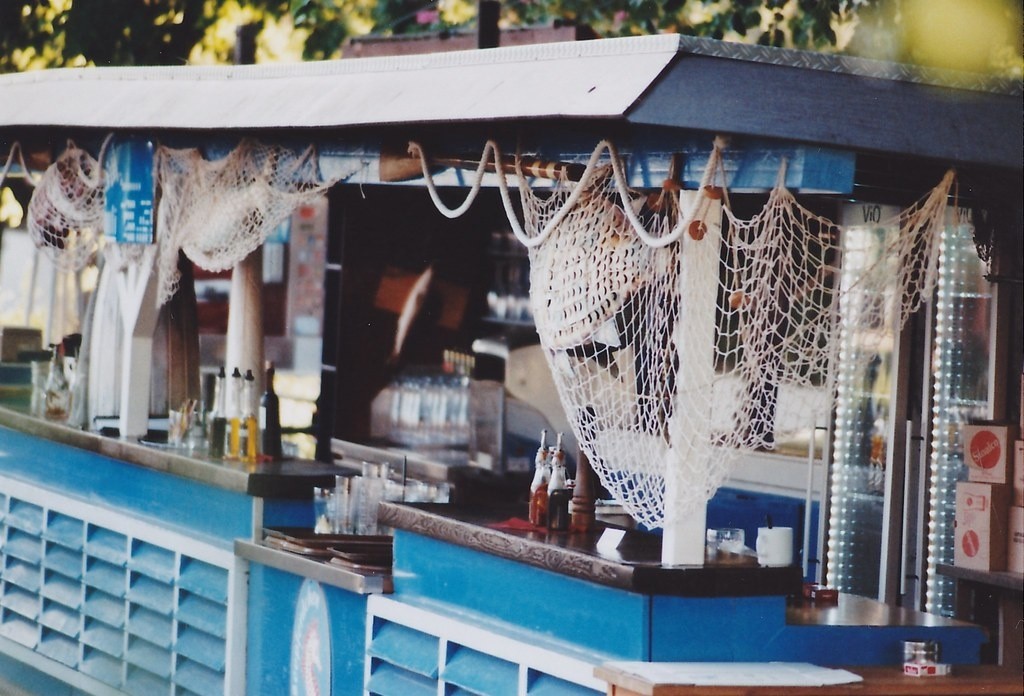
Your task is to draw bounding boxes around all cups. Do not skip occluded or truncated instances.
[313,461,450,536]
[706,528,745,565]
[29,357,74,422]
[756,526,794,568]
[169,408,207,451]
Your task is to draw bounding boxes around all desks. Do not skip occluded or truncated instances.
[594,665,1024,696]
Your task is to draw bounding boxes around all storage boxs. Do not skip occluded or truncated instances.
[953,373,1024,575]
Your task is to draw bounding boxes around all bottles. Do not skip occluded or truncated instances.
[388,376,469,430]
[243,369,255,462]
[227,366,239,458]
[547,433,566,528]
[208,367,227,459]
[261,361,284,457]
[529,430,551,526]
